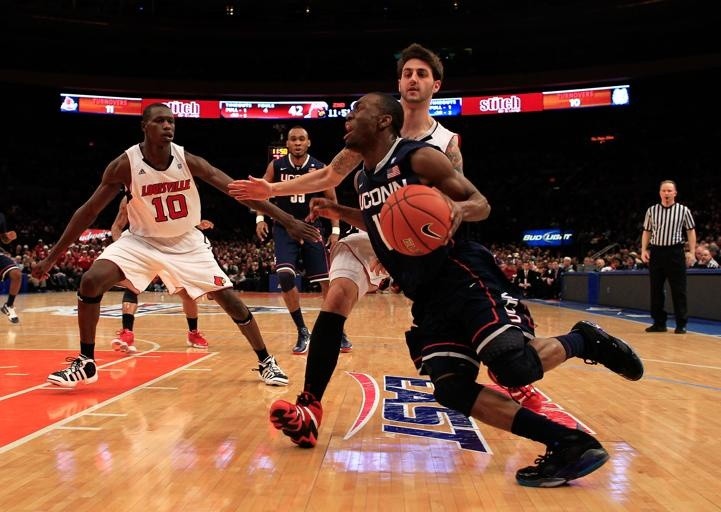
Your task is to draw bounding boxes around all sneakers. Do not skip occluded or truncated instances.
[292,328,310,355]
[515,428,610,489]
[339,332,353,352]
[269,391,324,450]
[487,368,542,413]
[569,320,644,382]
[46,353,99,389]
[187,330,208,349]
[1,302,19,323]
[109,328,137,353]
[256,355,290,388]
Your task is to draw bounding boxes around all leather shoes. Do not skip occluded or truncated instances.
[644,320,667,334]
[673,325,687,334]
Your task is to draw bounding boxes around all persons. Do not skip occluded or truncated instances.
[547,262,564,299]
[682,233,693,255]
[305,91,643,487]
[701,250,718,269]
[227,44,545,448]
[255,127,354,354]
[0,214,22,322]
[640,180,696,334]
[14,230,112,292]
[516,261,537,299]
[584,240,643,271]
[492,243,564,266]
[213,234,278,278]
[691,203,720,251]
[576,226,615,246]
[110,184,214,353]
[540,260,555,296]
[31,103,324,389]
[694,247,707,269]
[684,253,693,268]
[559,257,574,276]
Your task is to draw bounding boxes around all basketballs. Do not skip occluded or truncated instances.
[380,185,454,257]
